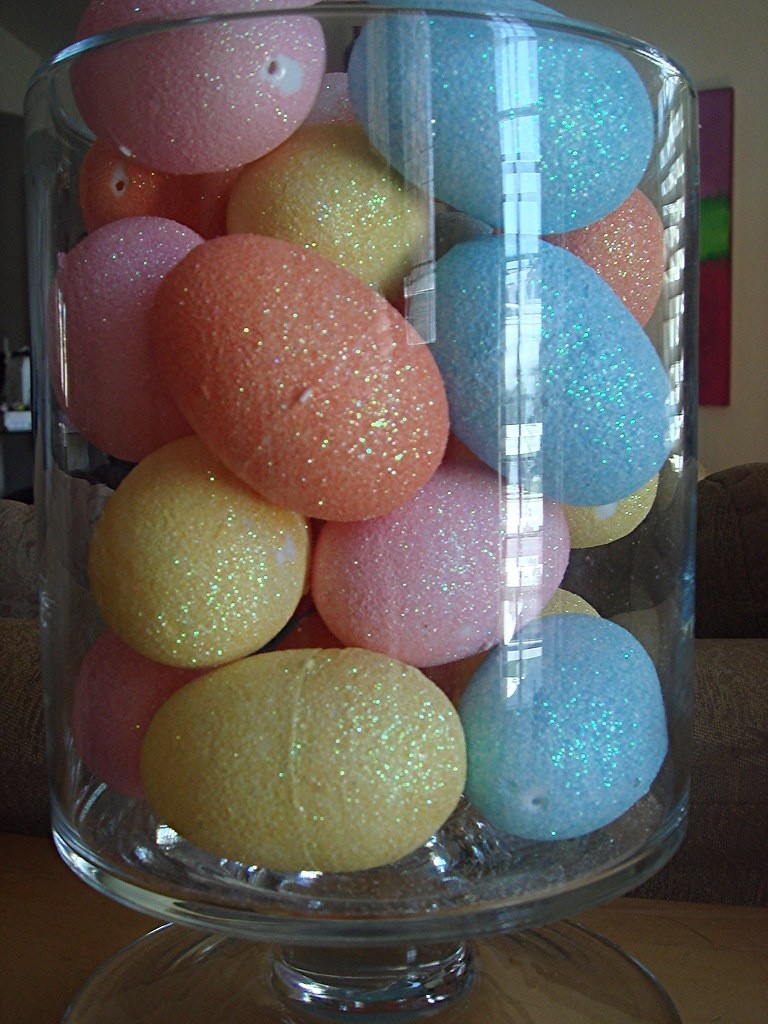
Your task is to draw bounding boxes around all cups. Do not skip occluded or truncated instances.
[19,11,696,1024]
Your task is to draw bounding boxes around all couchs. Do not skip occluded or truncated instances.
[562,463,766,907]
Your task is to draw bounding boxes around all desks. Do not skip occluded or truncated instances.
[1,827,768,1024]
